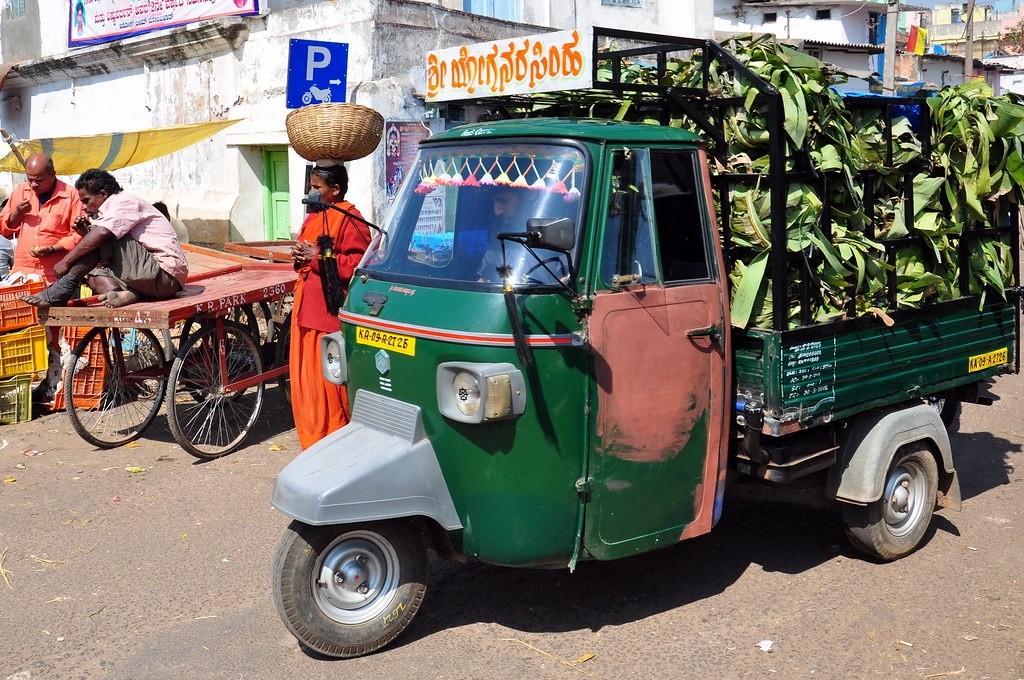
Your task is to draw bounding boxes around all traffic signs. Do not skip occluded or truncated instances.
[285,40,348,113]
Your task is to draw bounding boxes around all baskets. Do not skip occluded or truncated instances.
[286,104,385,160]
[0,276,109,423]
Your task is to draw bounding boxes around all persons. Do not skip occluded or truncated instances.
[0,152,189,353]
[475,180,551,283]
[289,164,373,440]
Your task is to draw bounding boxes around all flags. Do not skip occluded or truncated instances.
[905,24,926,55]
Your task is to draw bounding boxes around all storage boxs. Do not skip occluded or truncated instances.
[0,276,109,425]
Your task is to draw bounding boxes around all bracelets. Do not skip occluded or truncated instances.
[50,245,56,255]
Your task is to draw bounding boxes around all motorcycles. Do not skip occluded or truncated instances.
[269,38,1024,661]
